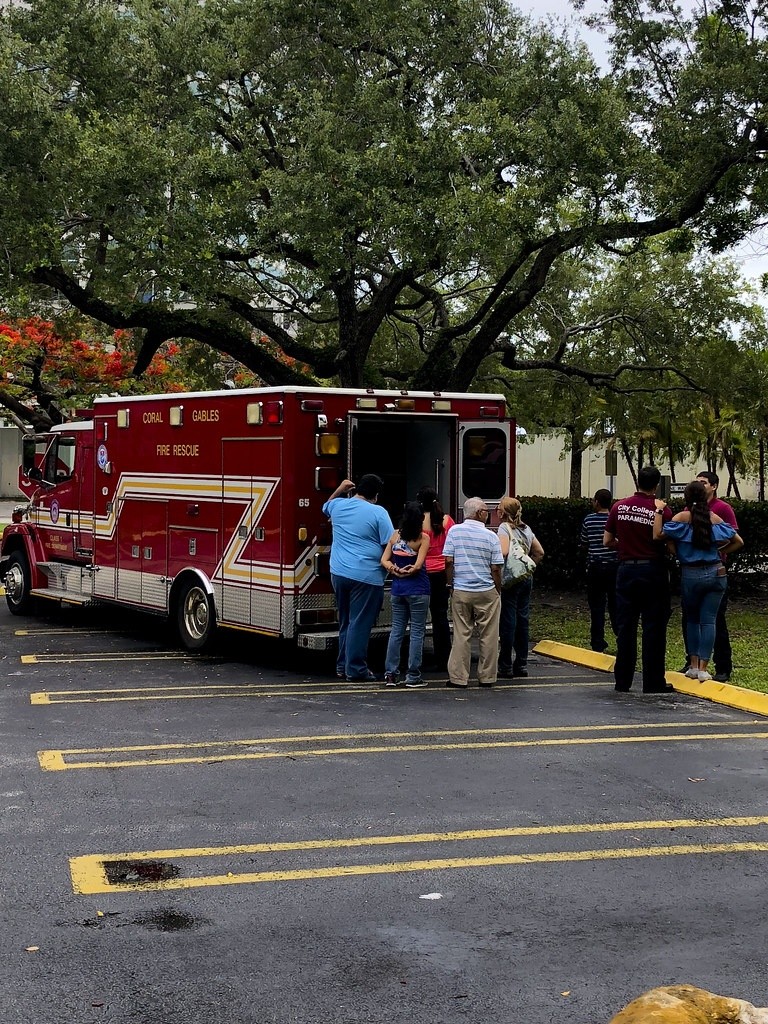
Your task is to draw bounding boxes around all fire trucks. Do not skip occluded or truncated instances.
[0,385,529,660]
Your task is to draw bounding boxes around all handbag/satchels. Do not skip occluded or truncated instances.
[500,522,536,587]
[339,668,373,679]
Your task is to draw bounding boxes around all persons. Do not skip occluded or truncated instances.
[496,496,545,680]
[322,474,395,683]
[579,488,619,652]
[380,499,429,688]
[653,481,744,681]
[414,484,456,672]
[603,467,676,693]
[442,497,504,688]
[677,471,739,682]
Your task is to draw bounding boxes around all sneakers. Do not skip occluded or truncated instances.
[385,673,400,686]
[406,680,429,688]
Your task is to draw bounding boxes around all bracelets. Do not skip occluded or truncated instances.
[656,509,664,514]
[445,582,452,589]
[395,566,400,573]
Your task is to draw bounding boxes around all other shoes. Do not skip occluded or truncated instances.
[713,671,730,682]
[514,665,529,677]
[644,683,673,693]
[698,671,713,681]
[346,673,379,682]
[685,666,698,678]
[497,665,513,678]
[677,662,692,673]
[614,684,629,692]
[445,681,468,688]
[479,682,493,688]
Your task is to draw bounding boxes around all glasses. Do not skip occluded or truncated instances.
[496,508,502,512]
[481,509,491,513]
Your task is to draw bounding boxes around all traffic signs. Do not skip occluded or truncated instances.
[670,482,690,494]
